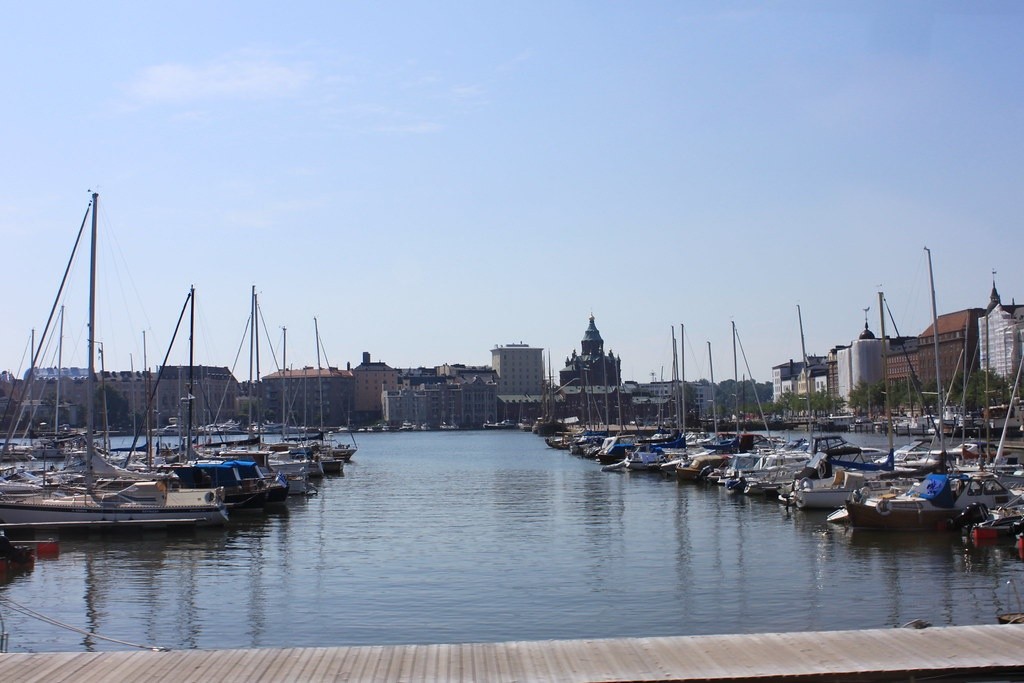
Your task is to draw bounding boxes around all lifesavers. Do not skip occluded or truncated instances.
[799,477,813,489]
[257,479,263,489]
[875,499,893,515]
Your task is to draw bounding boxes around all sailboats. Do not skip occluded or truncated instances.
[483,247,1023,567]
[0,188,461,527]
